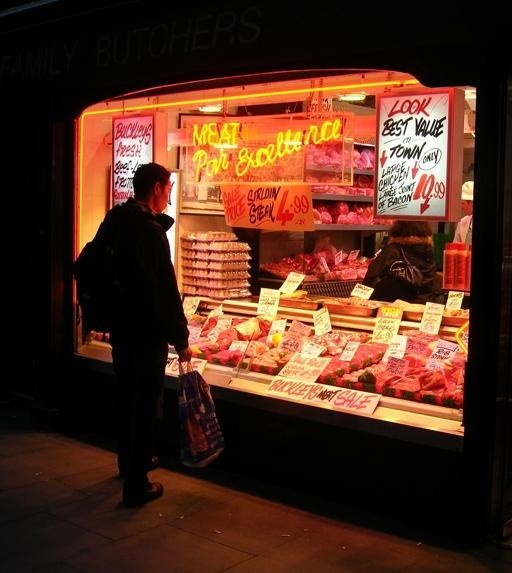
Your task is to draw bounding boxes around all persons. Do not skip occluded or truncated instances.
[361,220,435,303]
[444,200,473,309]
[76,164,192,505]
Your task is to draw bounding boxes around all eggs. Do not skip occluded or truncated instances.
[182,231,250,299]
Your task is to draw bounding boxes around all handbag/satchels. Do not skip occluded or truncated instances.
[178,357,226,469]
[373,245,427,299]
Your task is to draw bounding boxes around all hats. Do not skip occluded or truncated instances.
[460,181,474,201]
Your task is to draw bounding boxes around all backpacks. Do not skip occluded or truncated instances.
[75,216,153,333]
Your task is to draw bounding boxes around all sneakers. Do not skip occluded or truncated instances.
[119,453,160,479]
[125,480,163,506]
